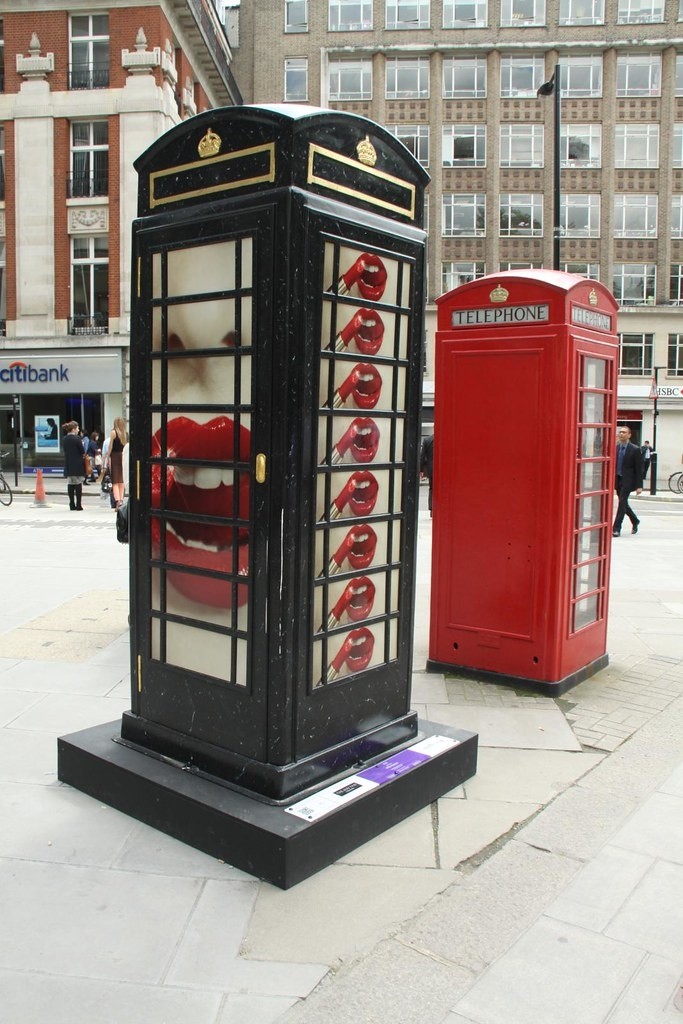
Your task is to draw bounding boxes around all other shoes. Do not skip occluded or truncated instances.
[83,480,90,485]
[115,500,123,512]
[69,502,76,510]
[75,502,83,510]
[88,479,96,482]
[613,531,619,537]
[632,520,640,534]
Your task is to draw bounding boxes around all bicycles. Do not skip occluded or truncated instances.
[0,452,13,506]
[667,472,683,495]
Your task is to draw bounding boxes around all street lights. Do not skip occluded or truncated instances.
[537,63,564,270]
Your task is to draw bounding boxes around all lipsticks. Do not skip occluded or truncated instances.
[323,314,363,353]
[322,369,361,410]
[320,424,359,466]
[318,533,355,579]
[326,259,365,296]
[319,479,357,523]
[316,586,354,634]
[315,638,353,687]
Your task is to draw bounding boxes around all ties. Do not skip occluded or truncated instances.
[617,446,626,475]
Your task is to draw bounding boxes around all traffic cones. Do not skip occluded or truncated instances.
[28,468,52,508]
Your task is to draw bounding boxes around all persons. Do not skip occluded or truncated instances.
[77,427,112,485]
[640,440,654,480]
[612,426,643,537]
[310,236,410,688]
[145,236,264,689]
[419,433,434,518]
[101,416,129,511]
[42,417,58,440]
[62,421,86,511]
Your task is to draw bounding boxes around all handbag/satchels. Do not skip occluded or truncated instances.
[95,449,103,469]
[101,465,113,492]
[83,454,93,476]
